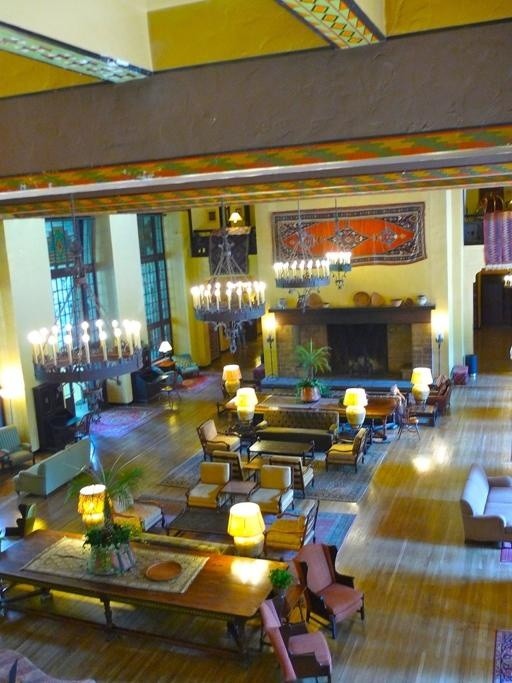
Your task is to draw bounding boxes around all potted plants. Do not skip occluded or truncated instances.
[67,448,157,576]
[291,337,333,403]
[272,569,292,596]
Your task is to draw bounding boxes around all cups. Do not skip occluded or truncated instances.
[391,299,402,307]
[417,294,427,305]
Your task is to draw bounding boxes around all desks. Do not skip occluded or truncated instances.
[214,479,256,508]
[1,529,288,663]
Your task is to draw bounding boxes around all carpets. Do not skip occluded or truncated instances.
[77,405,164,440]
[501,540,512,562]
[492,628,512,683]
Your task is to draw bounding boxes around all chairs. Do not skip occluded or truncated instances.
[113,364,455,444]
[3,503,38,538]
[256,497,320,549]
[248,464,292,516]
[323,428,367,475]
[183,460,230,519]
[291,542,365,640]
[172,354,199,380]
[267,455,317,500]
[258,601,332,683]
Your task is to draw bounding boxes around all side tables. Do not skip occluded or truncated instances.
[260,586,307,670]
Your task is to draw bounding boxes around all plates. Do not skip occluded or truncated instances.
[371,292,384,306]
[145,561,182,582]
[353,291,369,307]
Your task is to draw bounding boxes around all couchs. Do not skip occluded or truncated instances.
[0,424,35,475]
[13,436,90,500]
[460,463,512,543]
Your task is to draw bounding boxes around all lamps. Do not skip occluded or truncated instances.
[74,484,113,530]
[503,274,512,287]
[474,187,512,271]
[26,194,145,386]
[186,205,267,355]
[324,199,352,291]
[269,201,335,313]
[228,211,242,225]
[226,501,267,555]
[158,340,174,362]
